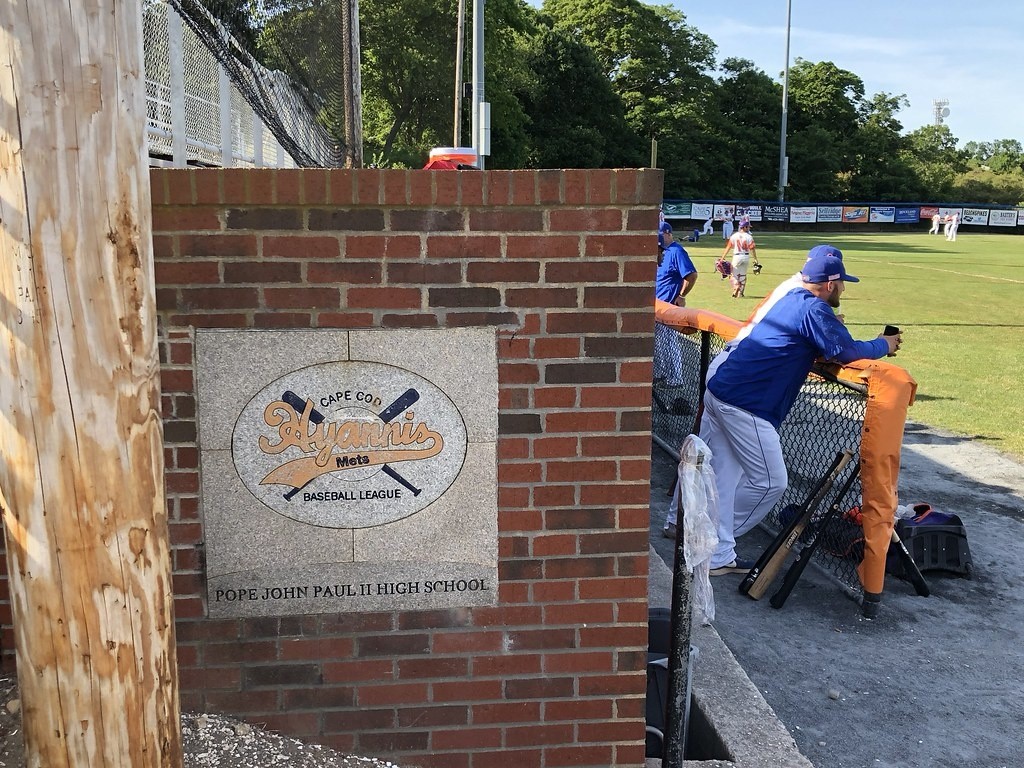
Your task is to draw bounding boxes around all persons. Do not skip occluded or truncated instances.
[722,207,735,240]
[720,221,758,297]
[662,244,904,576]
[652,209,699,390]
[929,211,960,242]
[738,210,753,234]
[699,215,721,236]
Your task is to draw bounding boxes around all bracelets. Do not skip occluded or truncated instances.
[679,295,686,299]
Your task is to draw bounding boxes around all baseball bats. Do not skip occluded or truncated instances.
[739,448,860,610]
[892,528,931,597]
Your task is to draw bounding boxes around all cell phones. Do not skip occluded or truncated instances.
[883,326,899,337]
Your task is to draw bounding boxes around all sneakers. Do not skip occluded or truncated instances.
[709,556,758,576]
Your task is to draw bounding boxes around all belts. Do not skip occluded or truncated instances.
[734,253,749,255]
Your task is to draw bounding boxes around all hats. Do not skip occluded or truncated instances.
[659,222,672,234]
[957,211,960,215]
[802,255,860,283]
[936,212,940,214]
[658,233,670,251]
[944,211,947,214]
[739,222,749,228]
[806,244,842,263]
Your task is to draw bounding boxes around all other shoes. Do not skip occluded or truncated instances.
[664,522,677,538]
[951,239,955,241]
[732,292,738,297]
[929,230,931,235]
[739,293,744,297]
[946,239,950,241]
